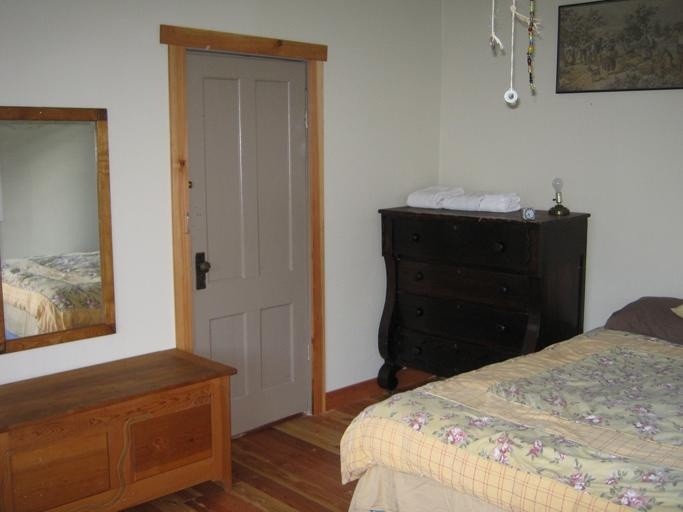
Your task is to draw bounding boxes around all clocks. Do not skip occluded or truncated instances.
[519,206,535,223]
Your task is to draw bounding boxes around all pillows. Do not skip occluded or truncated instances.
[603,295,682,344]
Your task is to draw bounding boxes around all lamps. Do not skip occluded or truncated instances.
[544,176,570,216]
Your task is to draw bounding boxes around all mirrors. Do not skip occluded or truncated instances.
[0,106,116,354]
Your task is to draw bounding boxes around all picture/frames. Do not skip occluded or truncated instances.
[554,1,683,92]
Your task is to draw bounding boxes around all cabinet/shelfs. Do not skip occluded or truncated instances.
[376,210,591,391]
[0,369,237,511]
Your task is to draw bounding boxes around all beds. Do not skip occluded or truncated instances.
[0,252,103,338]
[340,326,683,512]
